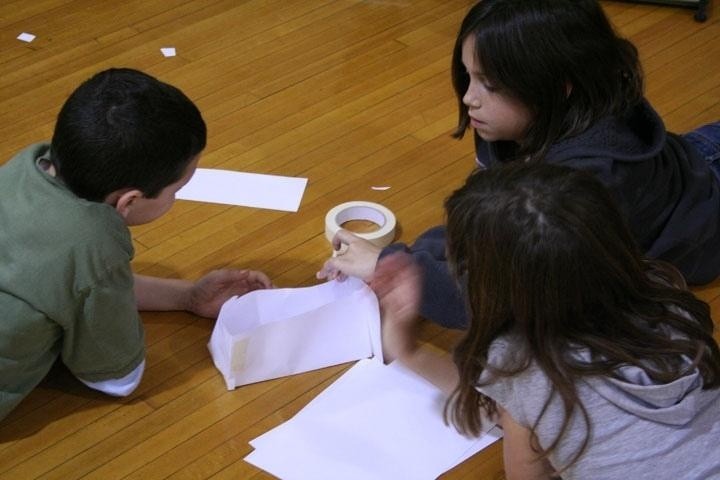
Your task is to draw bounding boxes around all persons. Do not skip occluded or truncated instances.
[370,156,719,479]
[314,1,719,330]
[0,68,278,426]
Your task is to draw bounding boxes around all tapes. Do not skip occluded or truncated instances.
[325,201,396,249]
